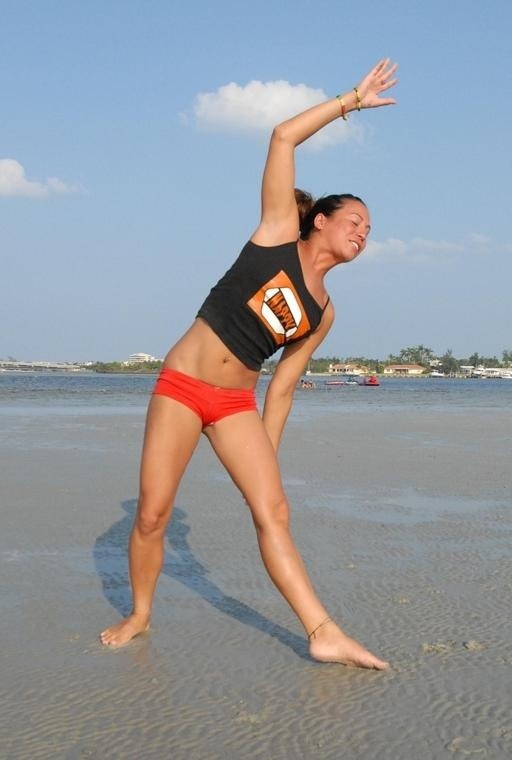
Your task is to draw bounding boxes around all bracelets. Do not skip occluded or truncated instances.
[353,87,362,112]
[336,93,348,123]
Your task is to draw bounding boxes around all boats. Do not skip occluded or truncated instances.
[469,366,512,379]
[344,378,358,385]
[324,379,344,385]
[428,369,444,378]
[359,377,380,386]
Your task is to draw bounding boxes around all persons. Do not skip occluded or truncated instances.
[300,379,314,389]
[98,51,399,672]
